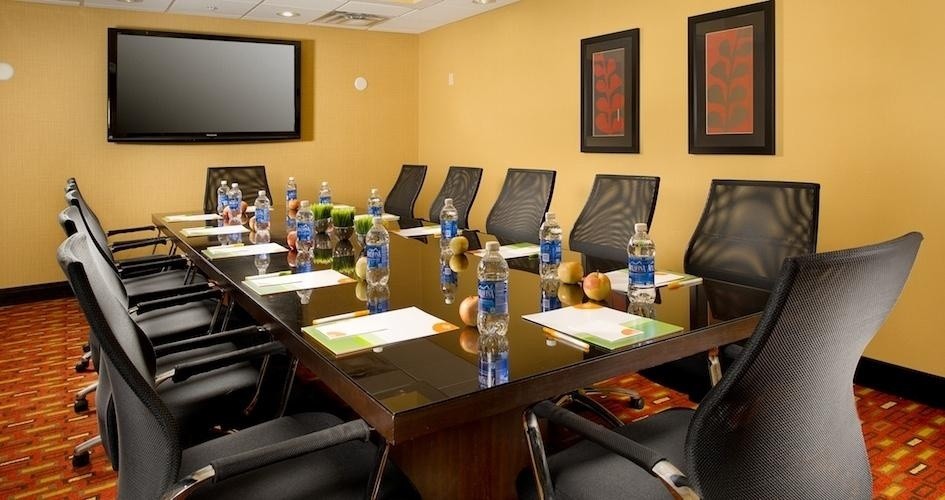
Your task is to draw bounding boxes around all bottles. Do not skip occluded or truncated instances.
[254,251,270,277]
[368,189,383,218]
[626,221,657,305]
[627,302,658,352]
[476,240,511,337]
[294,251,315,304]
[439,249,459,305]
[541,281,562,347]
[478,334,509,391]
[538,212,562,281]
[213,175,333,255]
[437,198,459,252]
[365,284,390,354]
[367,216,390,287]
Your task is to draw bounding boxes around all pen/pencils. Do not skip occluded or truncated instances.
[262,271,290,276]
[196,225,213,228]
[314,310,369,324]
[542,328,590,349]
[669,279,702,288]
[225,243,247,246]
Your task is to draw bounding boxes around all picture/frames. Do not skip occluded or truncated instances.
[578,28,641,155]
[686,1,777,156]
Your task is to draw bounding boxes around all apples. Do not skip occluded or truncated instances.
[249,216,256,230]
[241,213,248,224]
[224,206,229,217]
[242,201,247,213]
[459,296,478,326]
[449,236,468,254]
[223,218,228,225]
[355,257,367,280]
[460,327,479,353]
[287,251,297,266]
[557,262,583,284]
[289,209,297,217]
[449,255,468,271]
[289,200,299,209]
[287,231,297,249]
[249,231,255,243]
[583,272,611,300]
[558,284,583,305]
[356,280,367,300]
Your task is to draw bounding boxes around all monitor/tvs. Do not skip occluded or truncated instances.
[108,28,301,142]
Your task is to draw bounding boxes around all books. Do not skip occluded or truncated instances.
[297,305,461,358]
[466,239,543,261]
[200,242,291,261]
[355,212,401,223]
[245,205,275,214]
[520,300,687,353]
[601,267,698,292]
[179,224,252,238]
[162,213,223,223]
[242,267,358,295]
[388,224,465,238]
